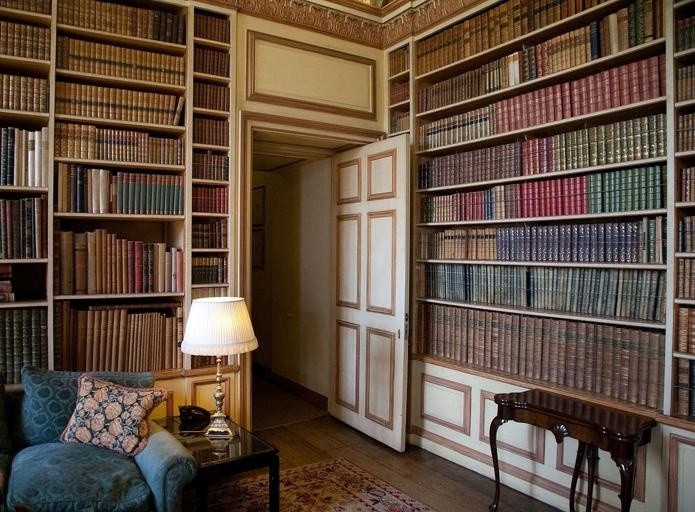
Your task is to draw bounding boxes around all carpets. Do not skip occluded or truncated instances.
[198,457,437,511]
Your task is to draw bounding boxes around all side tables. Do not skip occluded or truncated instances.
[154,408,282,512]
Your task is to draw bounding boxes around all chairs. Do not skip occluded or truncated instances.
[1,382,199,511]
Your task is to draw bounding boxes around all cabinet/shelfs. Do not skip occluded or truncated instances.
[380,1,693,432]
[2,1,249,380]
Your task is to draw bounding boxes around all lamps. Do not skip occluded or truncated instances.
[182,294,259,443]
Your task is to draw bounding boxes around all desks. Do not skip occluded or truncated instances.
[479,390,657,512]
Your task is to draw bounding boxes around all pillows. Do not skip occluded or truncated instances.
[16,357,167,458]
